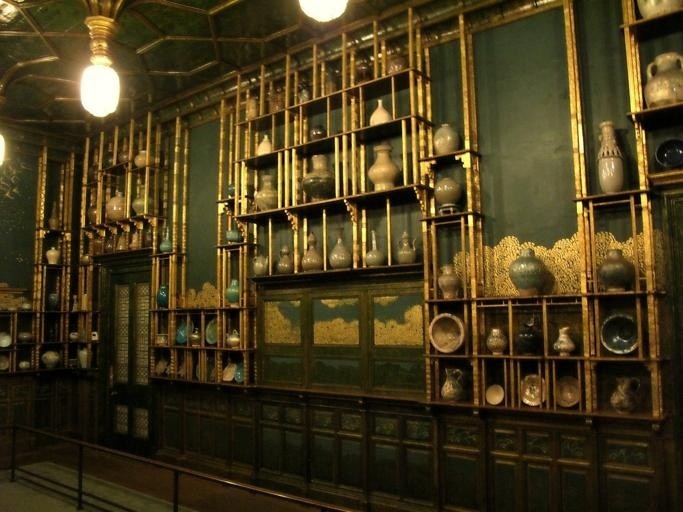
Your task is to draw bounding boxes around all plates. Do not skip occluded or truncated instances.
[204,316,217,345]
[482,373,581,409]
[0,355,9,370]
[0,332,12,348]
[154,356,245,383]
[175,318,193,344]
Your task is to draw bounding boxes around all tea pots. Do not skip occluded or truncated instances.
[607,375,643,413]
[225,329,241,349]
[250,252,269,277]
[439,366,467,400]
[393,229,419,264]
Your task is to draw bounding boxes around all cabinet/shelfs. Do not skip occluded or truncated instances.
[37,115,97,370]
[1,286,98,371]
[422,190,661,420]
[154,252,250,385]
[91,114,187,254]
[566,1,681,197]
[219,14,477,278]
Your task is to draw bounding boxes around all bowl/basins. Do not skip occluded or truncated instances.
[426,313,467,354]
[599,313,639,353]
[655,137,683,169]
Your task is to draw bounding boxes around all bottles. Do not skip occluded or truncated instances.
[365,230,384,268]
[595,119,624,193]
[597,249,634,291]
[157,286,168,308]
[277,244,293,274]
[237,51,408,128]
[300,231,322,272]
[255,134,271,156]
[310,126,326,140]
[508,248,545,297]
[636,1,682,20]
[17,136,157,371]
[432,123,460,156]
[515,323,539,356]
[254,175,277,210]
[225,279,239,307]
[553,326,575,357]
[485,328,508,355]
[329,228,351,269]
[190,327,200,346]
[437,264,461,300]
[159,225,172,252]
[642,51,683,109]
[433,176,463,208]
[302,154,335,202]
[367,143,400,192]
